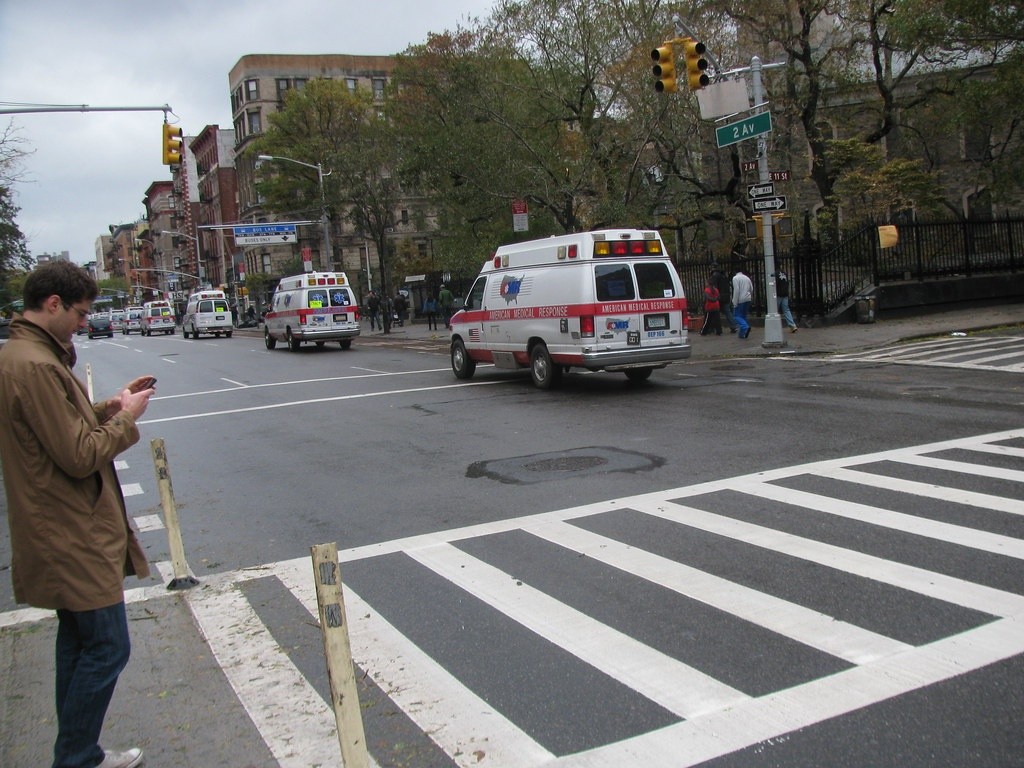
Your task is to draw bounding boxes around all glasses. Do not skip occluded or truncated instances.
[57,297,91,322]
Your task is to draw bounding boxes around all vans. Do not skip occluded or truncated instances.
[119,307,143,335]
[88,310,124,330]
[449,229,692,390]
[180,290,233,339]
[137,301,176,336]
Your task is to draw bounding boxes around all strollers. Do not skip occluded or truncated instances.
[392,306,403,328]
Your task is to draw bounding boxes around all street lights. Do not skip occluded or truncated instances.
[162,231,202,291]
[363,205,371,292]
[258,156,331,272]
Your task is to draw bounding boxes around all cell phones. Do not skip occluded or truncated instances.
[141,377,157,390]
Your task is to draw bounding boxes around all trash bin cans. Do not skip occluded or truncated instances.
[854,295,876,324]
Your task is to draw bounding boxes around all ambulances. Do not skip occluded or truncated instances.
[264,271,360,351]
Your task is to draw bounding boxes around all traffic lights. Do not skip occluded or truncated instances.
[774,216,794,237]
[684,41,709,91]
[162,124,183,165]
[745,219,762,240]
[651,44,677,93]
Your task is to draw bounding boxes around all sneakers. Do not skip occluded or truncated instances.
[90,747,144,768]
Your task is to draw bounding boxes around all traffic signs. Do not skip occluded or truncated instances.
[752,196,787,213]
[233,224,297,247]
[747,182,775,200]
[769,171,788,181]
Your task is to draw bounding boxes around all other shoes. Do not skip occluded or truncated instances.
[745,327,751,338]
[791,327,797,332]
[729,329,737,333]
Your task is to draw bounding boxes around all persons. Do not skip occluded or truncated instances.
[710,262,737,333]
[247,304,256,319]
[232,307,239,327]
[732,265,753,338]
[0,260,156,768]
[697,278,722,335]
[423,290,438,330]
[438,285,454,328]
[385,292,406,329]
[367,291,382,331]
[774,258,798,333]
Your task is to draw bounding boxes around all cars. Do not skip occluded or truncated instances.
[88,319,113,339]
[77,320,91,335]
